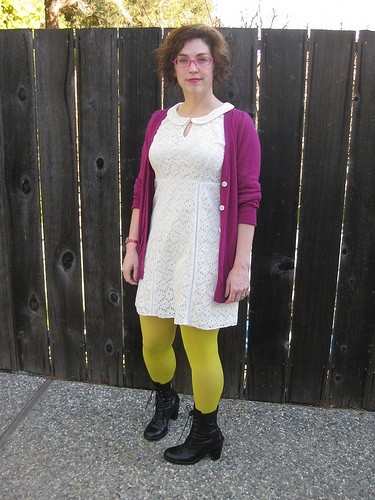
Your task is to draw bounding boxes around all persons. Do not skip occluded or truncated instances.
[123,23,262,465]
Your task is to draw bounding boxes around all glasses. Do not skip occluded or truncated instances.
[171,55,215,67]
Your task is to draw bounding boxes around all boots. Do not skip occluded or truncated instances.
[164,402,225,465]
[144,377,180,441]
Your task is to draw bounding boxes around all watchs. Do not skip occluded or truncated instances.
[125,237,138,245]
